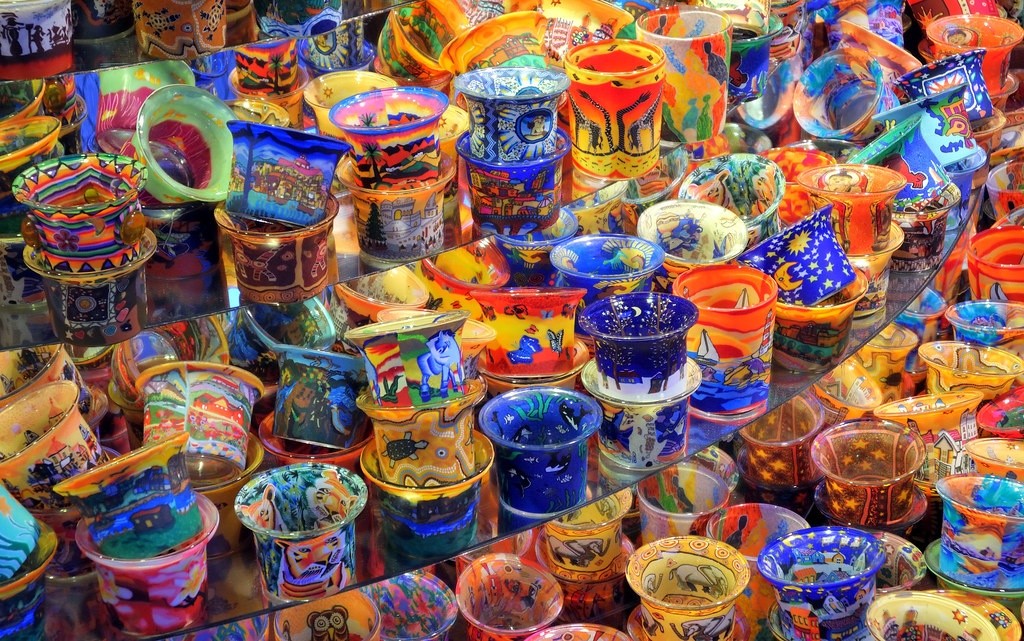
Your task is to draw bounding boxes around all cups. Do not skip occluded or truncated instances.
[0,0,1024,641]
